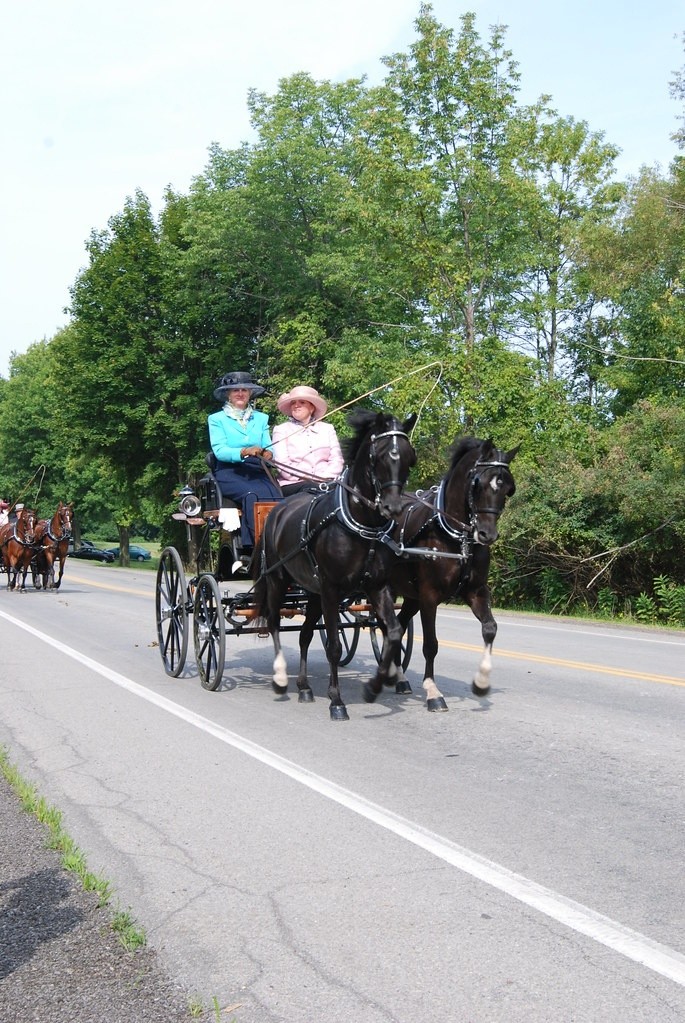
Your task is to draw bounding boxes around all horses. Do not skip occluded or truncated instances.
[0,499,74,594]
[246,408,419,722]
[389,435,523,712]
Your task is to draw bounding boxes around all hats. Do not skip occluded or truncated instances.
[277,386,326,419]
[214,371,265,402]
[15,503,24,512]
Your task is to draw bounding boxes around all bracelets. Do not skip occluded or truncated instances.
[241,448,247,458]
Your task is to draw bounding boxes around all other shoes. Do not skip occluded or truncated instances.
[241,555,251,566]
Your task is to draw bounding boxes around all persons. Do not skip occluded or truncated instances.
[14,504,24,521]
[270,385,345,496]
[0,499,9,529]
[207,372,282,552]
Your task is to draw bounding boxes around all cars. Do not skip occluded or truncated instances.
[68,549,115,563]
[106,546,152,562]
[66,539,95,554]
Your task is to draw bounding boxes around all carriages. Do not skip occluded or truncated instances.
[154,409,525,720]
[0,500,74,593]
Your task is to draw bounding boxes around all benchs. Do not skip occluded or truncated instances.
[198,452,244,517]
